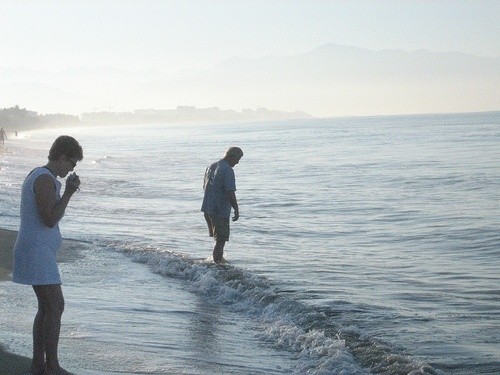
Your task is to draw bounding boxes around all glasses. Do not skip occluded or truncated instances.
[67,159,76,168]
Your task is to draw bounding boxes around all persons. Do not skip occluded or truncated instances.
[12,134,85,375]
[200,146,244,263]
